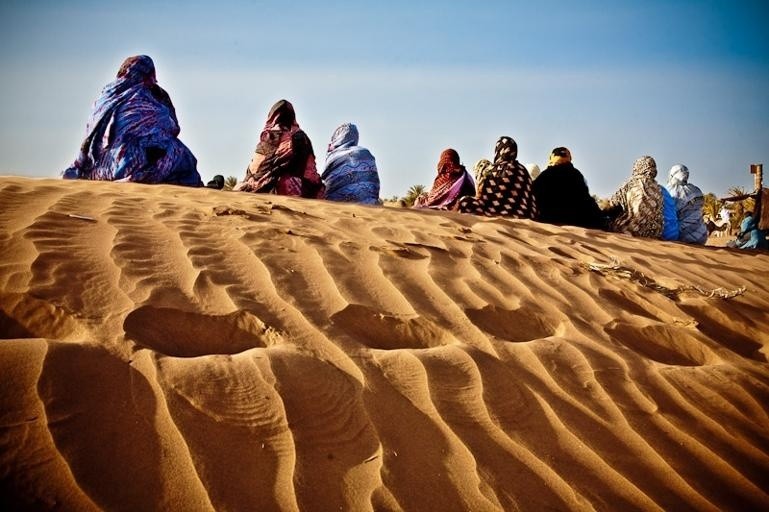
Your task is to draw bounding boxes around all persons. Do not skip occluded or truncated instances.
[734,211,765,249]
[660,184,679,241]
[240,100,326,199]
[532,147,607,231]
[411,149,476,211]
[455,136,539,221]
[321,122,380,206]
[602,156,664,238]
[59,55,203,185]
[667,164,707,245]
[528,164,541,181]
[473,158,493,184]
